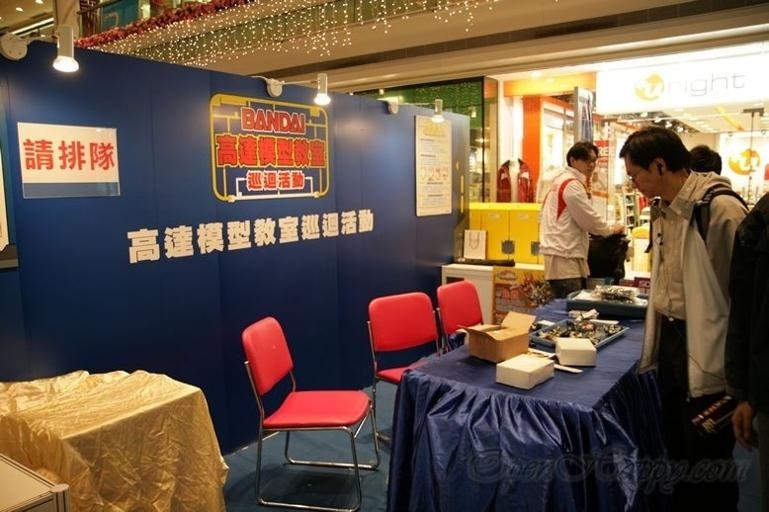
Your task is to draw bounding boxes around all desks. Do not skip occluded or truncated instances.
[387,297,661,512]
[0,453,70,511]
[0,367,230,512]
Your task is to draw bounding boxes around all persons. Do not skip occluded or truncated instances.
[539,140,628,302]
[686,145,722,174]
[618,127,753,512]
[726,190,768,510]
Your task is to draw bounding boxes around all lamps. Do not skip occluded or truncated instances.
[389,99,444,124]
[2,25,79,72]
[266,73,331,105]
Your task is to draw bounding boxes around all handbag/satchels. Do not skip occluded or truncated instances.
[585,229,627,278]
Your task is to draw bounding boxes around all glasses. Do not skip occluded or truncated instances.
[624,167,644,181]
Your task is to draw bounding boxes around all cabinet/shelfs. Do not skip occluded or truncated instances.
[470,202,547,265]
[624,192,637,232]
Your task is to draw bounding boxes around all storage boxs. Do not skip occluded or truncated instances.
[457,310,536,364]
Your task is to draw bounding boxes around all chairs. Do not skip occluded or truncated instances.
[240,316,381,511]
[367,292,439,445]
[436,281,483,352]
